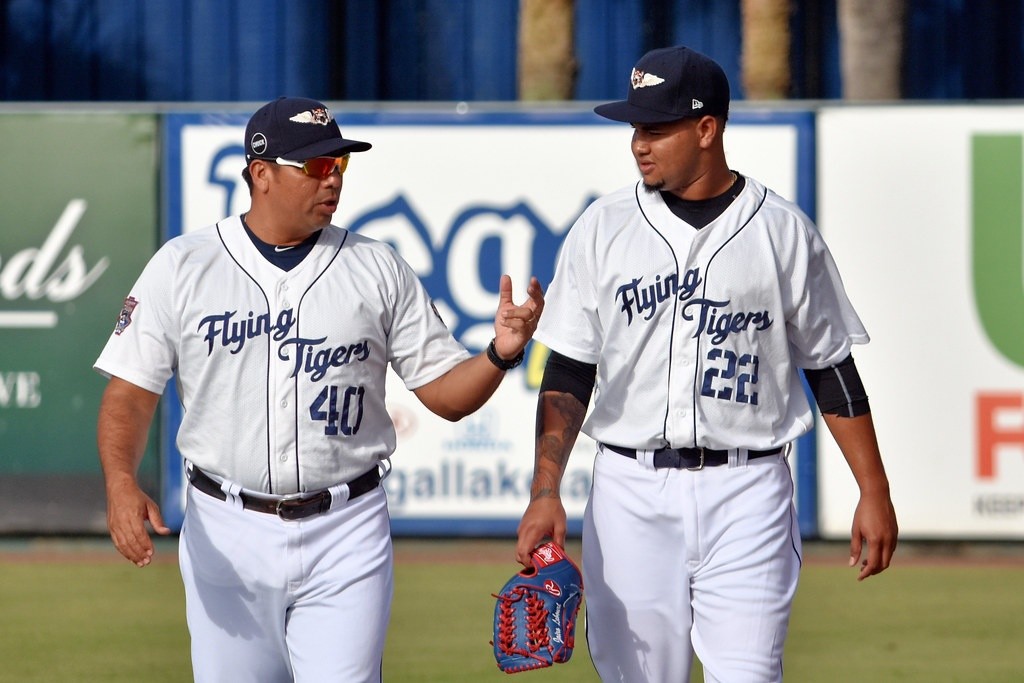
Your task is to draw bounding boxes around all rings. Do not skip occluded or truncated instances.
[527,314,536,323]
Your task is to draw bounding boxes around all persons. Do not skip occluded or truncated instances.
[515,44,899,683]
[91,97,545,683]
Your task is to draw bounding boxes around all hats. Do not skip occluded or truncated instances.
[593,45,730,125]
[244,96,373,164]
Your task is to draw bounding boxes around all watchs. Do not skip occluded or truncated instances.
[487,336,524,371]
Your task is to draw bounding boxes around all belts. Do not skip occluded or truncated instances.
[602,439,782,471]
[185,462,380,523]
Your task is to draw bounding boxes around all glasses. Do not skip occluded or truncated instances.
[249,153,351,181]
